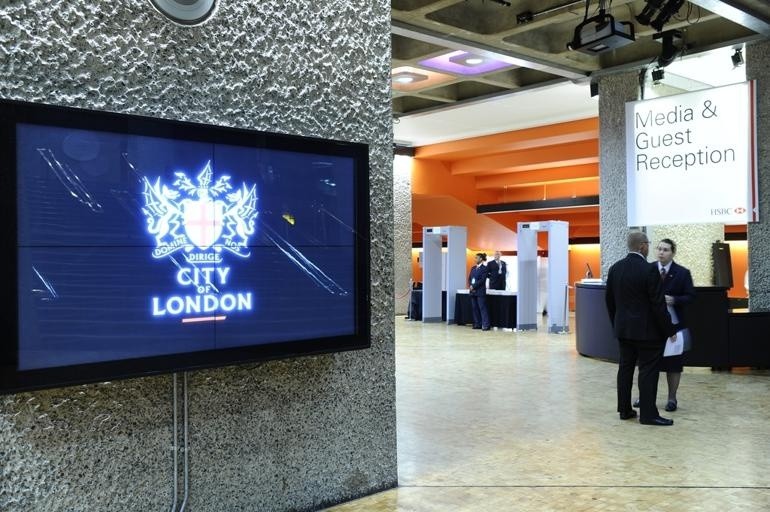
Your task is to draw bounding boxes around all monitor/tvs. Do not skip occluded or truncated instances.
[0,98,372,396]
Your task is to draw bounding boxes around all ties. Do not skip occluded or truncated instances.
[661,268,667,282]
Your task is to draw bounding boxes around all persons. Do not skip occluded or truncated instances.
[467,253,491,331]
[604,232,676,426]
[633,238,697,411]
[486,251,507,290]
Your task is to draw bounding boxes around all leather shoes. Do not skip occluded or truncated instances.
[473,325,490,330]
[665,399,678,411]
[634,398,640,407]
[640,416,674,426]
[619,410,637,420]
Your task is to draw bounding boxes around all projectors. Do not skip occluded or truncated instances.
[567,20,626,51]
